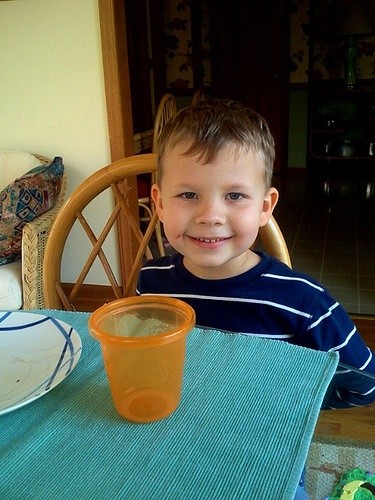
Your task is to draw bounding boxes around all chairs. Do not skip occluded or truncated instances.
[137,94,178,220]
[0,150,68,310]
[46,152,292,311]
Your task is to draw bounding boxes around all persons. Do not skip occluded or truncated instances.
[134,95,375,411]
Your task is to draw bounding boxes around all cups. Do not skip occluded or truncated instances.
[87,295,196,422]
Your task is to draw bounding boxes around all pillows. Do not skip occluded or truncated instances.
[0,156,65,268]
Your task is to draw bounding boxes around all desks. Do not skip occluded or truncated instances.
[0,311,339,500]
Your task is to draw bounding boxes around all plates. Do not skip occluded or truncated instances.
[0,311,83,415]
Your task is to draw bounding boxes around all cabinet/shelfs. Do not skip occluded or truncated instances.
[308,32,375,207]
